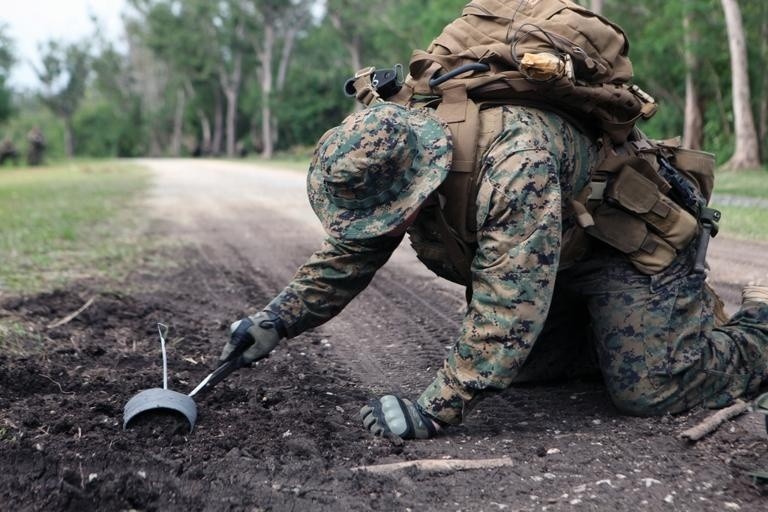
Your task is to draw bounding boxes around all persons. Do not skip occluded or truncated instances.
[0,137,20,166]
[28,126,44,166]
[219,99,768,440]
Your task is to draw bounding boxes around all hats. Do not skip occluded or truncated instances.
[306,101,454,241]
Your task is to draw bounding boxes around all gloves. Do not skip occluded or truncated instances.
[360,393,439,441]
[219,310,283,368]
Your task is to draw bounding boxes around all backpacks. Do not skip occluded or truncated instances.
[341,1,658,170]
